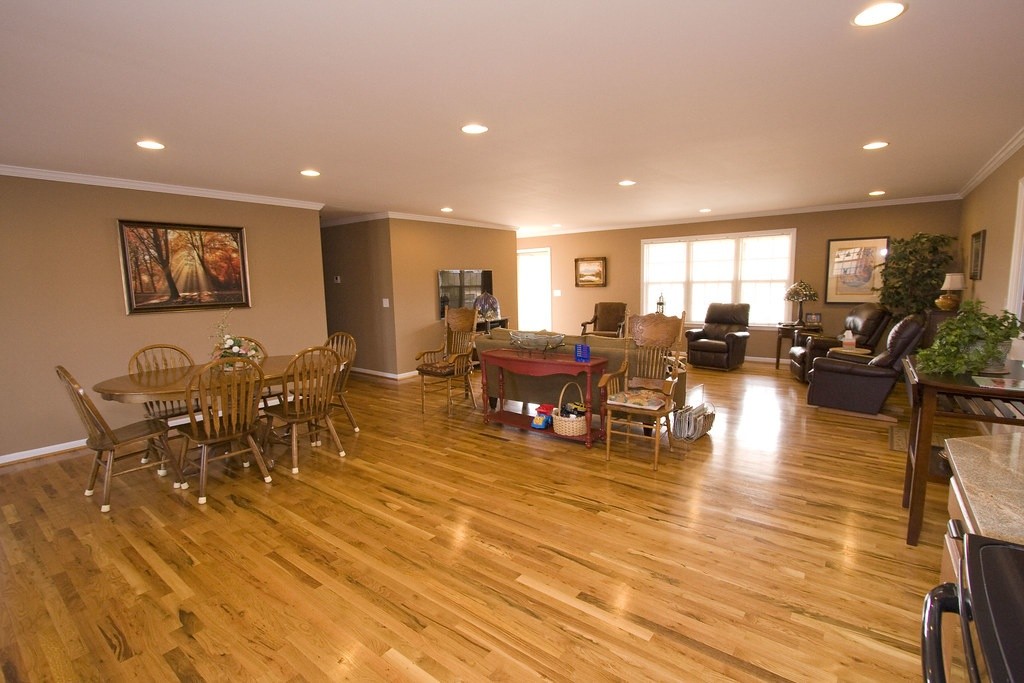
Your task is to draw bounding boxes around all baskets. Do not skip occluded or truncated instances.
[551,382,587,436]
[211,336,268,379]
[510,330,565,350]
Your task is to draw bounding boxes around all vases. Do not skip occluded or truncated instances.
[805,313,821,323]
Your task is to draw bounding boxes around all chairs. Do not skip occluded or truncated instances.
[54,364,189,512]
[580,301,627,337]
[599,310,686,473]
[787,302,928,414]
[684,303,750,372]
[129,332,361,505]
[414,304,480,417]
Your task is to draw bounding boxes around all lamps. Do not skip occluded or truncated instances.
[783,279,820,326]
[939,272,966,294]
[474,291,501,333]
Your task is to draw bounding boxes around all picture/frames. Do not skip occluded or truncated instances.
[116,218,251,317]
[574,256,606,287]
[824,235,888,305]
[969,229,985,280]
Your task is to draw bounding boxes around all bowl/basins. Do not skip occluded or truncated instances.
[508,331,565,350]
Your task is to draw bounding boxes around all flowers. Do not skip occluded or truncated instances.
[205,306,261,370]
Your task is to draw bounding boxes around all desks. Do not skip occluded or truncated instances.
[480,347,609,449]
[774,326,821,370]
[828,346,872,355]
[92,353,348,489]
[900,354,1023,546]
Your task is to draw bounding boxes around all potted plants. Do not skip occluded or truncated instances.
[914,298,1023,377]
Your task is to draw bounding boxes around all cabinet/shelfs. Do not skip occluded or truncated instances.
[472,315,510,367]
[932,432,1022,682]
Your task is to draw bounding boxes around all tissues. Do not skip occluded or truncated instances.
[842,330,856,350]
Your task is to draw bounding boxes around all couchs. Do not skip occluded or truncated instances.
[475,328,688,437]
